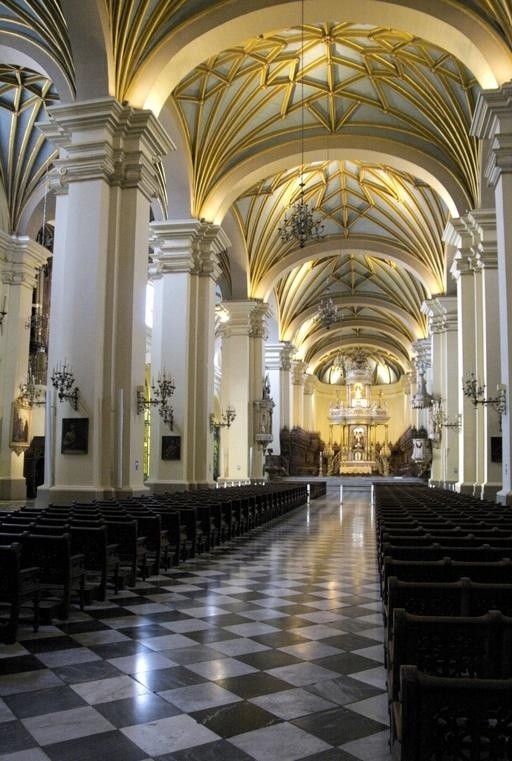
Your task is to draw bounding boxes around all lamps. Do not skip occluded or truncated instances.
[274,0,347,332]
[208,399,236,433]
[463,371,507,415]
[138,366,177,431]
[18,355,79,411]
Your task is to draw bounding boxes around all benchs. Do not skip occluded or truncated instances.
[0,481,329,645]
[372,481,512,761]
